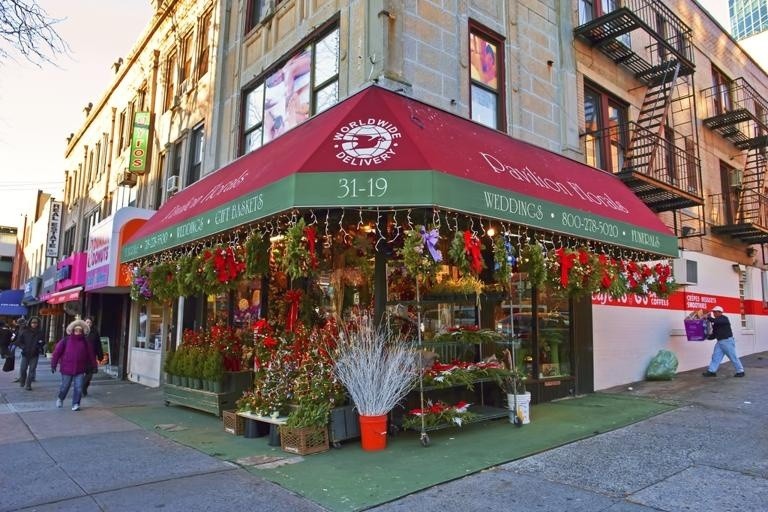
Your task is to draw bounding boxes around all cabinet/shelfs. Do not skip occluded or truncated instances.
[236,406,289,446]
[506,325,574,388]
[137,302,173,340]
[381,289,516,434]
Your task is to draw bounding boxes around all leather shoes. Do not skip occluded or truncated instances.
[26,386,32,391]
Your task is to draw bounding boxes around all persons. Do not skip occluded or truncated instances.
[83,318,104,398]
[264,47,311,144]
[1,315,45,392]
[217,289,261,321]
[702,306,746,378]
[49,318,98,412]
[469,44,498,83]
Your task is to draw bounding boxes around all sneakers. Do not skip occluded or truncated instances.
[703,371,716,377]
[735,372,744,377]
[72,403,82,411]
[56,398,63,408]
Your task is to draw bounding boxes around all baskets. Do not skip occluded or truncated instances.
[280,424,330,456]
[222,410,245,436]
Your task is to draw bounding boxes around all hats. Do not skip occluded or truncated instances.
[31,319,38,323]
[712,306,723,313]
[66,320,91,338]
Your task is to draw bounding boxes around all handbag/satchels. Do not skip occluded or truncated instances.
[3,342,16,371]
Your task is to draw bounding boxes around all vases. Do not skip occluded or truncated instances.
[43,340,56,361]
[223,354,241,372]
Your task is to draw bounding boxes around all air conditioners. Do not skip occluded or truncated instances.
[165,174,181,193]
[115,168,138,188]
[728,167,751,192]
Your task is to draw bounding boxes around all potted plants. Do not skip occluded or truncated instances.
[160,343,225,396]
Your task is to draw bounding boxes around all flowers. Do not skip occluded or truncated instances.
[180,321,246,359]
[239,314,340,405]
